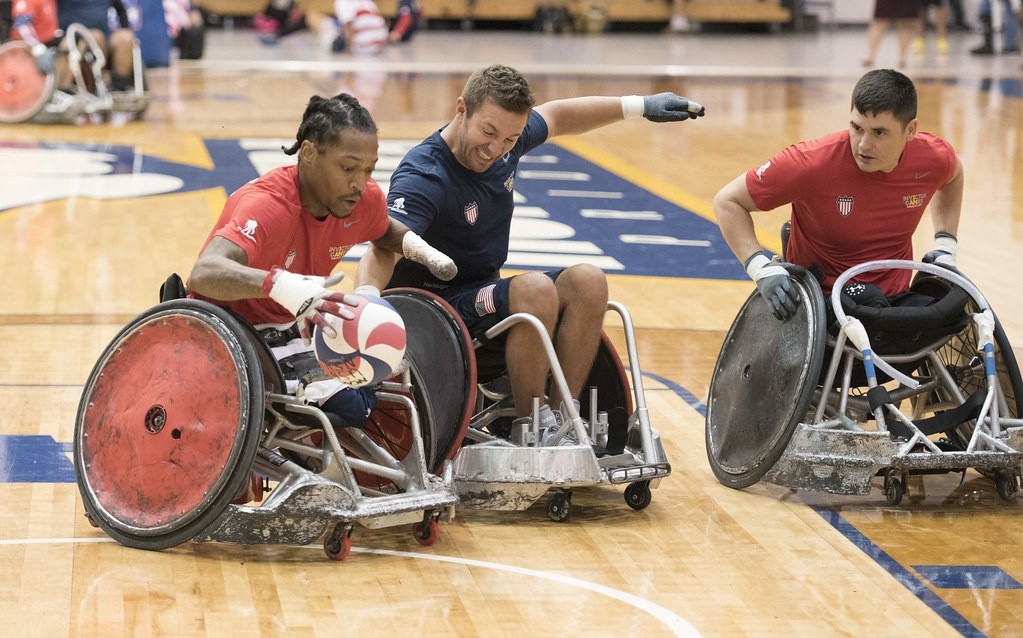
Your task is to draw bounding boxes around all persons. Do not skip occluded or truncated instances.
[256,1,422,49]
[354,65,705,445]
[712,69,964,319]
[187,92,458,423]
[862,0,1023,72]
[10,0,135,93]
[535,0,606,33]
[665,0,692,32]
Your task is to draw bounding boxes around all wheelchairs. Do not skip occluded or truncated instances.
[378,285,673,520]
[705,216,1023,507]
[0,22,152,127]
[72,273,459,564]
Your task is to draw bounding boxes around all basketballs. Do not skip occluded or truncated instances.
[312,292,407,390]
